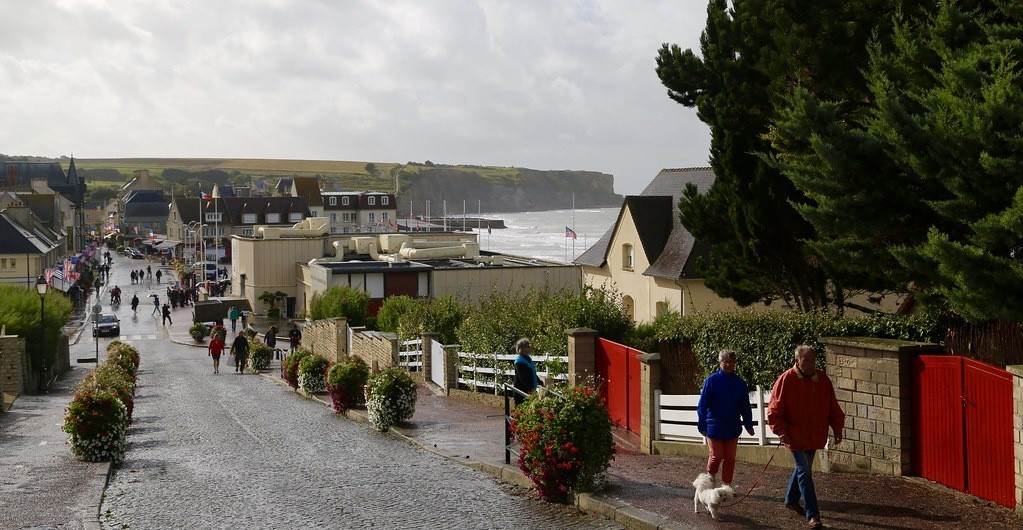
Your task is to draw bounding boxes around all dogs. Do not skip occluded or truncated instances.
[692,474,732,518]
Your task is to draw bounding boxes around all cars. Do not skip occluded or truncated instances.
[125,247,143,259]
[196,282,217,300]
[90,313,121,336]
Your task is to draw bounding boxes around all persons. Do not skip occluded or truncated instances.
[229,307,246,333]
[230,331,251,373]
[263,325,280,363]
[697,349,755,495]
[288,325,301,356]
[513,339,545,407]
[767,345,845,528]
[208,333,225,374]
[94,251,228,326]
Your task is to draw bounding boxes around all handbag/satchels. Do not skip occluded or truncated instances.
[290,335,300,343]
[226,352,237,367]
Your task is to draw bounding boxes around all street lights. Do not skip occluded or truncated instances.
[189,222,200,266]
[185,220,197,263]
[26,236,36,292]
[35,274,48,393]
[195,224,208,264]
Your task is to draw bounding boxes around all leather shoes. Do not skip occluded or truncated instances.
[807,516,823,529]
[784,502,805,515]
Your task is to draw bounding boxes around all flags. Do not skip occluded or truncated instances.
[379,213,425,233]
[199,190,212,201]
[43,222,154,287]
[488,222,491,233]
[566,226,576,239]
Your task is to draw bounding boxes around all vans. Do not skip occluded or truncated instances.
[193,262,216,279]
